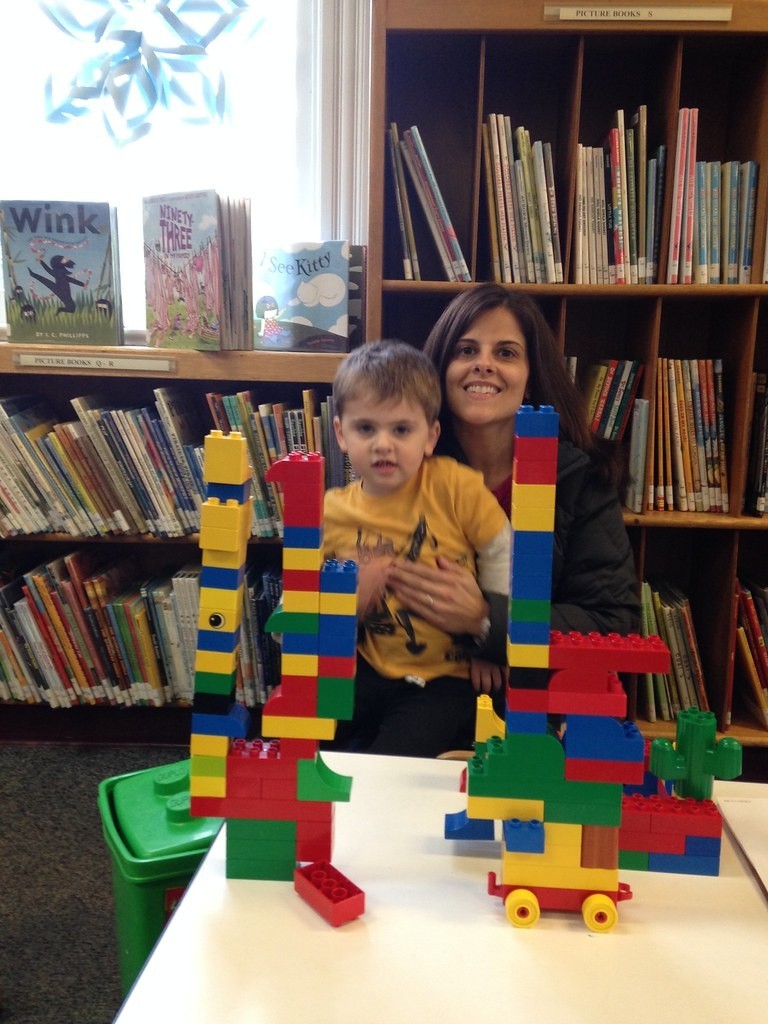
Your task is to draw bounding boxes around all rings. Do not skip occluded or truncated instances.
[425,593,436,608]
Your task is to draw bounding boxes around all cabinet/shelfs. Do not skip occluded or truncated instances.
[0,342,353,708]
[367,1,767,750]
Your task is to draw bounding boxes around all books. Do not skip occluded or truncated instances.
[667,107,761,285]
[569,356,767,521]
[385,116,469,282]
[574,104,669,287]
[0,197,126,348]
[256,240,367,353]
[641,581,768,731]
[482,111,565,283]
[0,387,360,711]
[140,188,255,352]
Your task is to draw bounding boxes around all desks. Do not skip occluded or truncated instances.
[113,752,768,1022]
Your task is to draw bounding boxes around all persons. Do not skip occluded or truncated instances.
[321,281,641,759]
